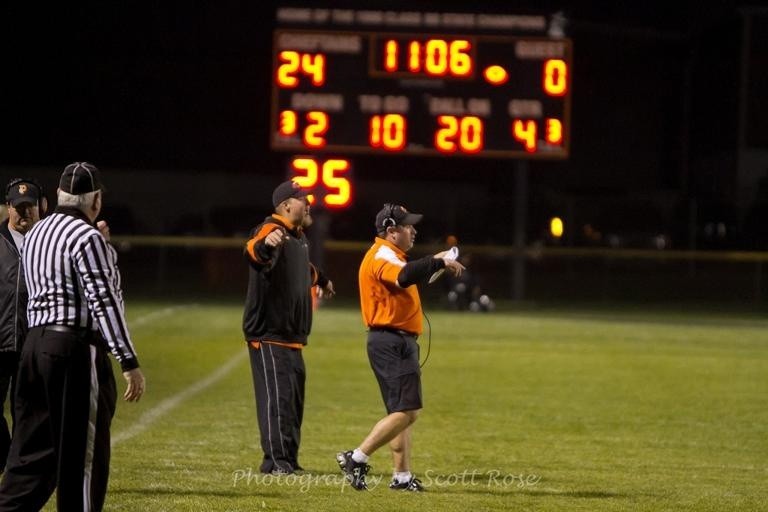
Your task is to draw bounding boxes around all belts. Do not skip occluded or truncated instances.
[42,323,87,336]
[370,327,416,336]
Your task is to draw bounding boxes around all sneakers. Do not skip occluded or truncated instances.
[389,477,423,492]
[336,450,373,491]
[5,175,42,208]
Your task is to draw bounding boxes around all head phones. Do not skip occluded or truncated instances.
[5,178,48,215]
[383,202,397,230]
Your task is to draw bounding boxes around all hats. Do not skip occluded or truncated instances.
[59,161,104,195]
[374,203,423,233]
[272,180,314,208]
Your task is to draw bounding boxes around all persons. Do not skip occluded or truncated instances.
[0,177,48,472]
[239,181,336,477]
[335,202,467,490]
[0,160,145,512]
